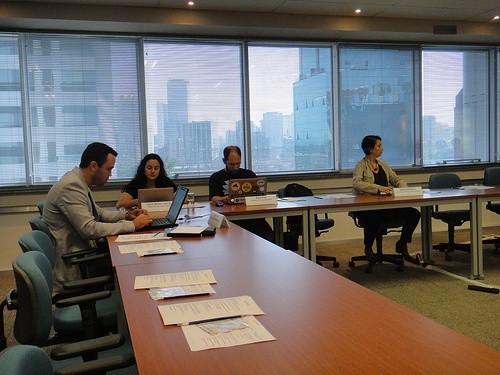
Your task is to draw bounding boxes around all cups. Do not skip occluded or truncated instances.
[187,193,195,210]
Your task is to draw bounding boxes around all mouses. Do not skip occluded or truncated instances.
[215,201,223,207]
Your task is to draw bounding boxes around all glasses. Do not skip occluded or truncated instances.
[145,164,160,170]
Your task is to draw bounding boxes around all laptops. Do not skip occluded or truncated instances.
[138,188,174,210]
[224,177,267,204]
[139,186,189,231]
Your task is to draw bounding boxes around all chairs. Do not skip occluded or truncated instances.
[349,211,405,273]
[482,167,500,255]
[0,201,136,375]
[430,174,471,255]
[278,188,339,268]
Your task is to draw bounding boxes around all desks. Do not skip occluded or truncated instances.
[103,184,500,375]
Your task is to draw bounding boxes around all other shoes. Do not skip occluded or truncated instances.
[365,248,375,261]
[396,241,410,259]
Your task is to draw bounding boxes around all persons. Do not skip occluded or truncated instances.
[116,153,189,210]
[41,142,154,293]
[352,135,421,264]
[208,145,274,242]
